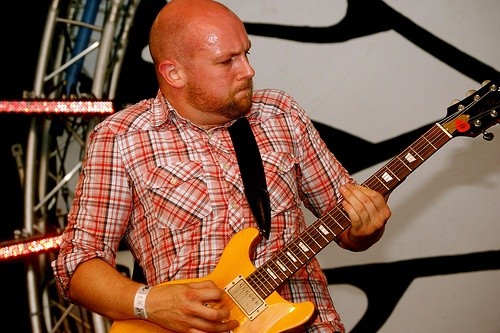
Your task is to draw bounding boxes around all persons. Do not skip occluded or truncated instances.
[51,0,392,333]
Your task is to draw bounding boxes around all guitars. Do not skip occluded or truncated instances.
[110,80,500,333]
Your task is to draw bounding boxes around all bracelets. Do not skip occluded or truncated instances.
[134,285,152,319]
